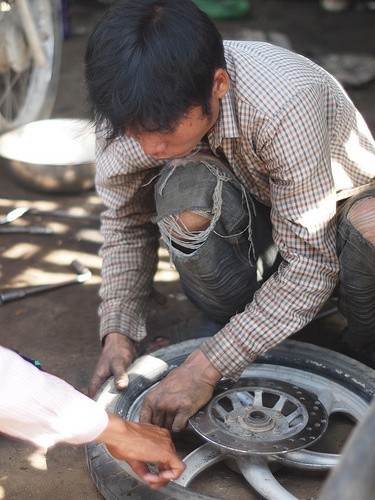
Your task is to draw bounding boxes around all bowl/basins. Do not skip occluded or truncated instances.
[0,119,96,194]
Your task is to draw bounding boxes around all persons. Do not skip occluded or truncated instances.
[73,1,375,434]
[0,344,186,491]
